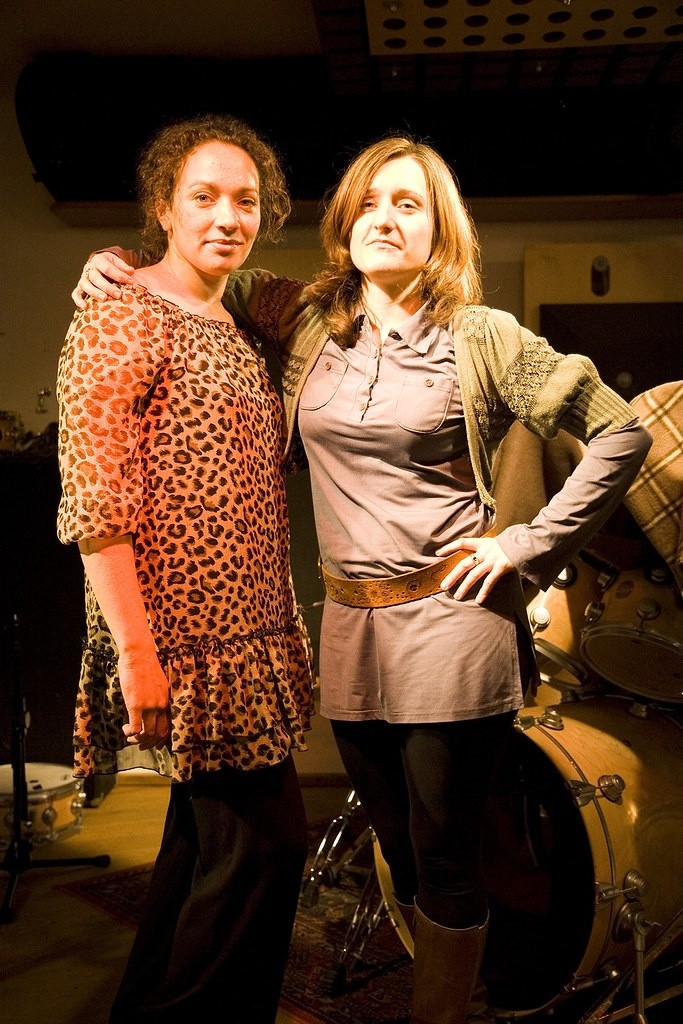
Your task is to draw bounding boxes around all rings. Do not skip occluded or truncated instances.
[470,554,480,565]
[85,266,93,280]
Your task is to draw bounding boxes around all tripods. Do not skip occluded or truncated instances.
[0,614,110,930]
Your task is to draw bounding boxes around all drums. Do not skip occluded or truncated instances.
[578,563,682,698]
[372,697,681,1024]
[522,550,610,698]
[1,761,85,850]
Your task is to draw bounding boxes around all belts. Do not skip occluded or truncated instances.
[316,525,501,608]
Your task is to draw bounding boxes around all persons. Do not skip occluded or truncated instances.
[74,137,653,1024]
[56,117,317,1024]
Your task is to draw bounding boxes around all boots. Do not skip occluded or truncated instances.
[391,891,415,943]
[403,905,490,1024]
